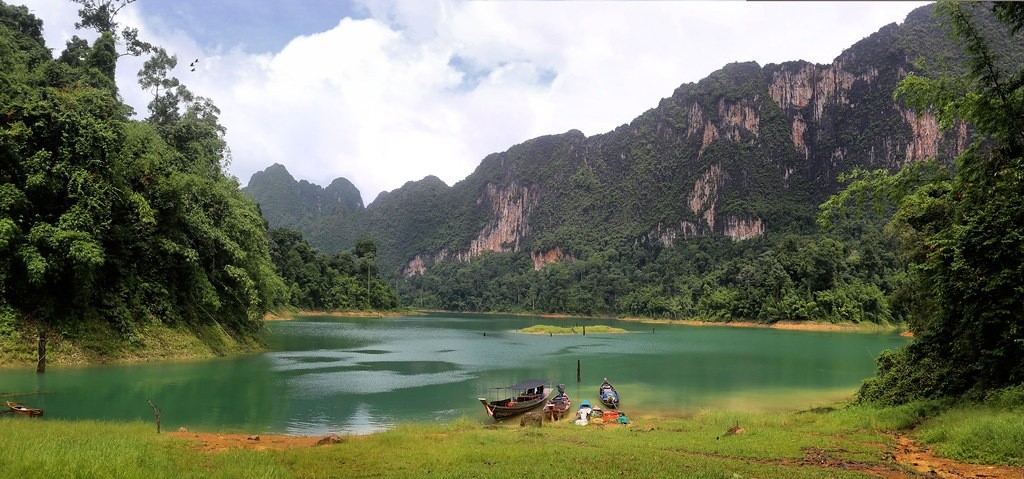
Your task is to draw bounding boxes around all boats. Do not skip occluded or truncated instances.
[6,400,43,417]
[599,377,620,409]
[576,399,629,426]
[543,384,570,421]
[477,378,554,422]
